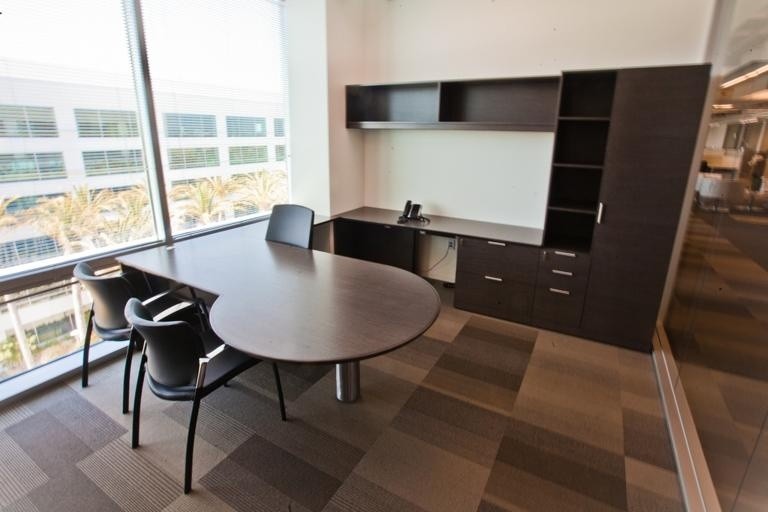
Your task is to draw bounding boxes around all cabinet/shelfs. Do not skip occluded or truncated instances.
[332,62,711,355]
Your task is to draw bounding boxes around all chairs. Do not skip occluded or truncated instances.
[123,297,288,496]
[698,175,747,218]
[264,204,315,250]
[73,260,203,415]
[695,170,724,205]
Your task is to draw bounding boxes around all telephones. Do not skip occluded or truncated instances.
[403,200,422,219]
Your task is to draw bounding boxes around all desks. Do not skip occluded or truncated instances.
[115,218,444,405]
